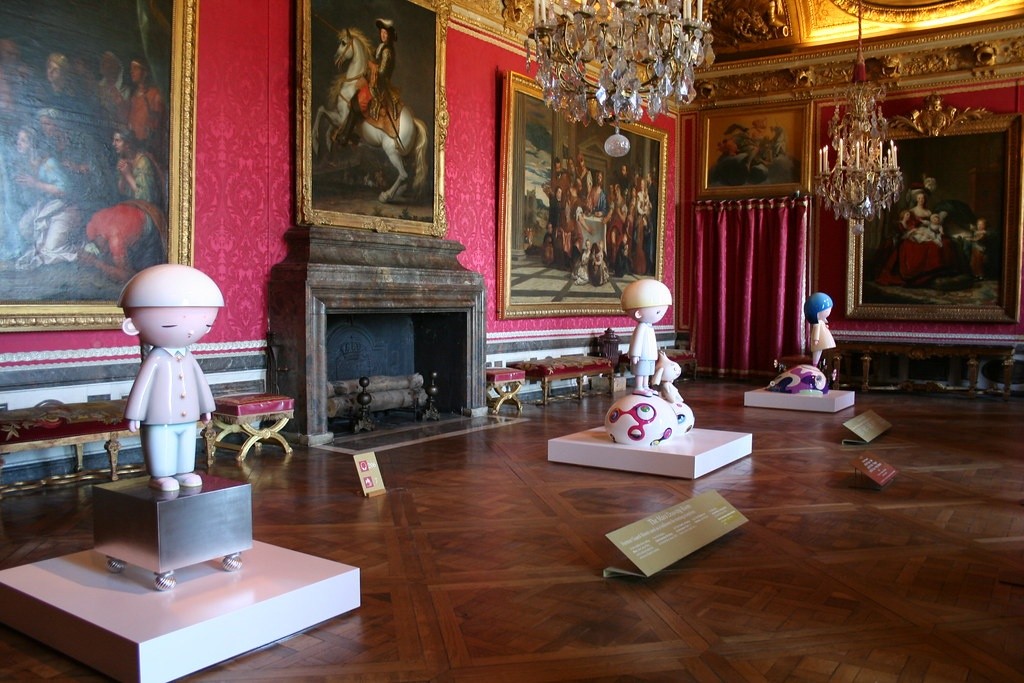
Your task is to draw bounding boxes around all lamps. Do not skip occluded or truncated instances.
[525,0,715,157]
[816,0,905,236]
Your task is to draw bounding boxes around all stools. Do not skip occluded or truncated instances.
[774,354,814,375]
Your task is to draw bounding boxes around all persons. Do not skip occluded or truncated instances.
[804,293,836,368]
[118,264,225,491]
[621,279,672,397]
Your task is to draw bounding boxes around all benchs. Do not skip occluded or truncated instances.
[0,399,216,499]
[509,355,615,407]
[208,393,294,462]
[486,367,526,416]
[619,349,697,388]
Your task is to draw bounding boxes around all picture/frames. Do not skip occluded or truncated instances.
[844,92,1024,324]
[295,0,452,237]
[696,95,814,202]
[496,69,669,320]
[0,0,199,333]
